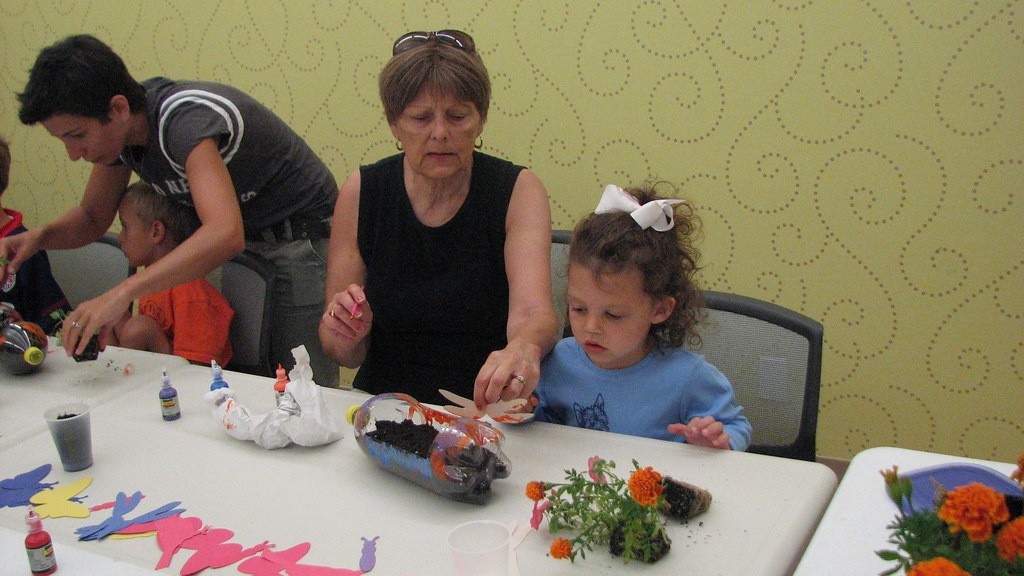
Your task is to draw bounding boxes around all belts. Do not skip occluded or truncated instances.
[247,209,333,242]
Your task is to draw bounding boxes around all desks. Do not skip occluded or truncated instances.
[0,366,840,576]
[0,334,190,450]
[791,447,1024,576]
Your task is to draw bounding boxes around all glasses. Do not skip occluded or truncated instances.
[392,29,483,62]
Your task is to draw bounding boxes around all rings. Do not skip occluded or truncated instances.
[71,321,80,328]
[510,372,526,382]
[330,303,337,316]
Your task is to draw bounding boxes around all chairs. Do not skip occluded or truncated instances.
[549,229,823,465]
[45,234,135,328]
[204,249,275,379]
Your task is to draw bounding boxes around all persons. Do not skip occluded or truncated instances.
[0,137,74,335]
[107,181,236,367]
[320,28,559,410]
[0,32,341,389]
[513,185,751,453]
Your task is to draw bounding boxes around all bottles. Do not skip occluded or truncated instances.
[158,369,181,421]
[274,363,290,407]
[345,393,513,504]
[25,504,57,576]
[210,360,229,406]
[0,303,49,375]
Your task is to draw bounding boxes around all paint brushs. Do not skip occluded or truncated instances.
[350,285,365,321]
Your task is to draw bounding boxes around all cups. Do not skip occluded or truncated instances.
[43,402,93,473]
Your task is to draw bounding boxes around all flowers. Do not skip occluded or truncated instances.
[525,458,713,563]
[873,465,1024,576]
